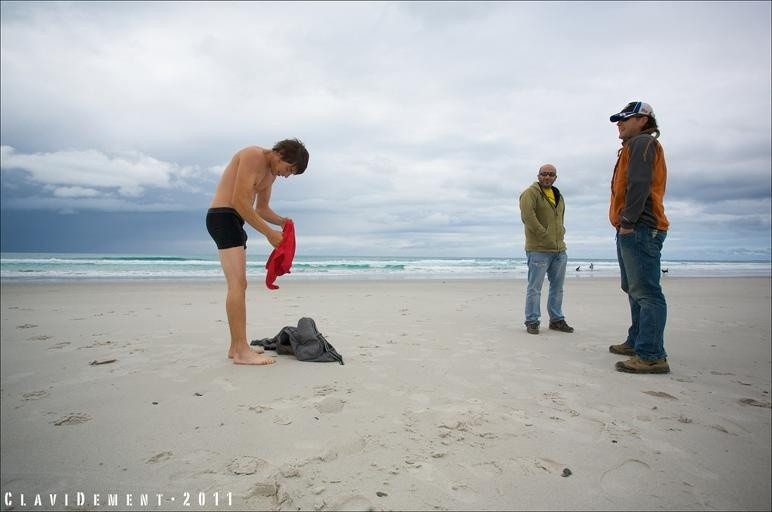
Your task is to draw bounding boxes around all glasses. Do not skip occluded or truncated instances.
[539,172,556,176]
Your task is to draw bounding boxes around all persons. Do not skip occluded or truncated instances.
[205,137,309,365]
[607,101,671,375]
[519,163,574,335]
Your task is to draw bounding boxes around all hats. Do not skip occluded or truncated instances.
[609,101,653,122]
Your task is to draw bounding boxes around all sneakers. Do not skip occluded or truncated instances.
[527,322,540,335]
[549,319,574,333]
[609,341,638,356]
[615,355,671,374]
